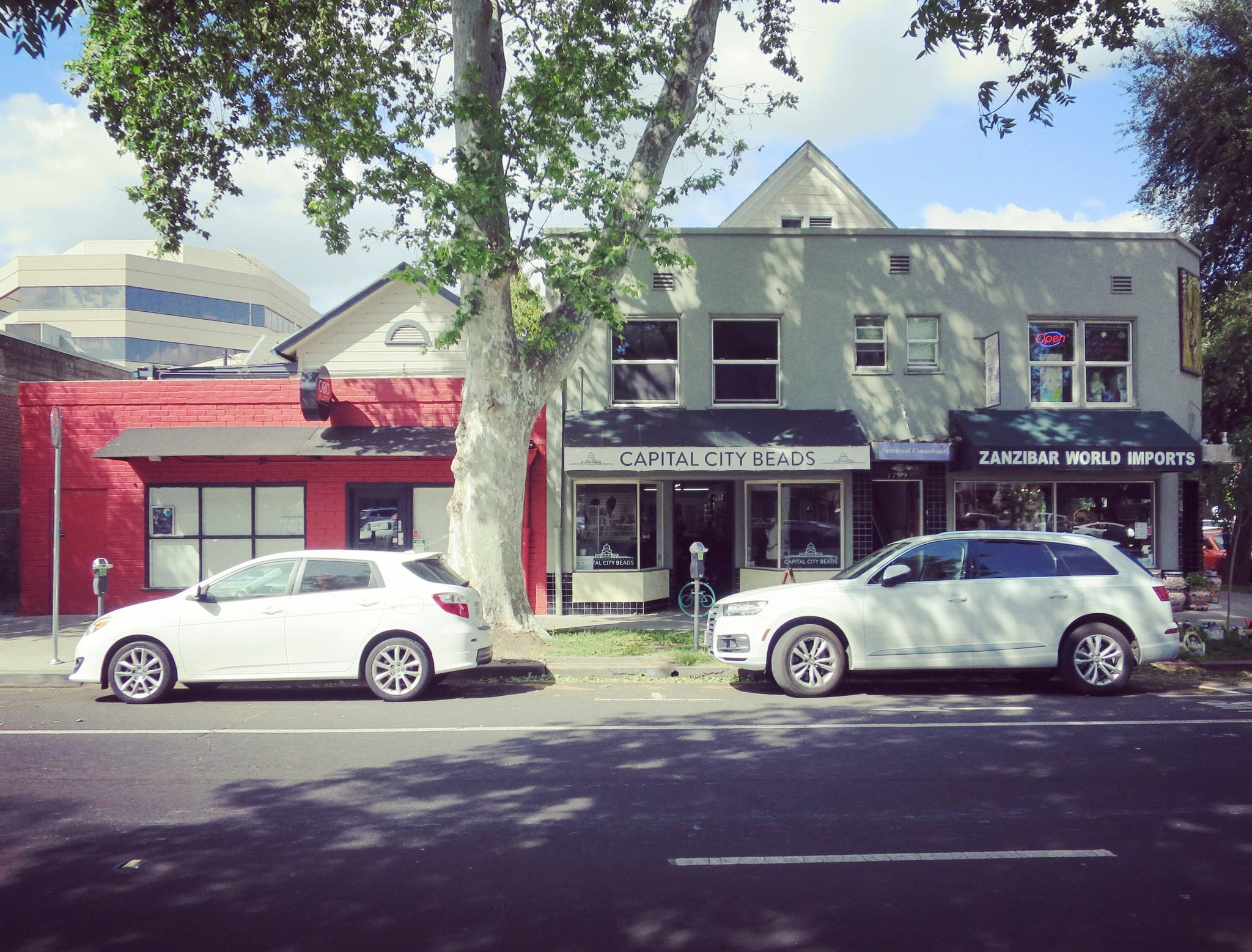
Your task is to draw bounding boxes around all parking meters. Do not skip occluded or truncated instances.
[690,542,708,650]
[91,558,113,618]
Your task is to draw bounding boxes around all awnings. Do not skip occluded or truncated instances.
[91,427,456,462]
[946,409,1203,472]
[564,409,871,474]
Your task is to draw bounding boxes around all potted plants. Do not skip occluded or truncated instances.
[1184,572,1211,605]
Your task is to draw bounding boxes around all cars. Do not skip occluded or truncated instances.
[359,507,397,542]
[703,529,1181,697]
[959,509,1151,557]
[67,549,494,704]
[1200,519,1233,583]
[752,518,841,568]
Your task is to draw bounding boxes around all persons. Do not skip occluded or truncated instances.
[1089,373,1106,402]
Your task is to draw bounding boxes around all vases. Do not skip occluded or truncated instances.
[1204,568,1222,603]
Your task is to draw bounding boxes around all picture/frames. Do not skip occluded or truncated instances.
[1178,266,1203,376]
